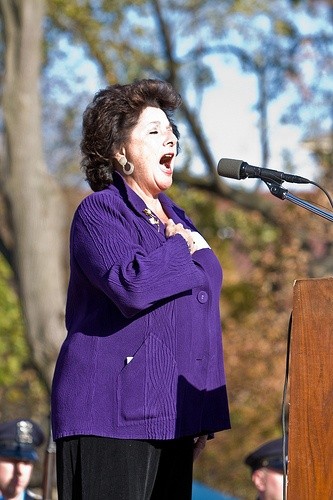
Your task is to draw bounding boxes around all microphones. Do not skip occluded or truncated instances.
[217,158,310,183]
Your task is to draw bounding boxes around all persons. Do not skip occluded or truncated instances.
[51,79,231,500]
[0,417,45,500]
[243,436,289,500]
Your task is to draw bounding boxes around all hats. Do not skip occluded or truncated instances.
[244,437,288,474]
[0,418,45,462]
